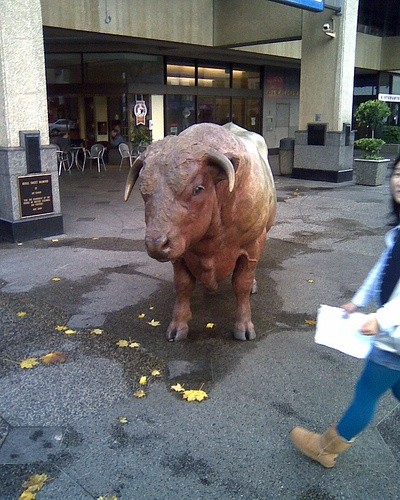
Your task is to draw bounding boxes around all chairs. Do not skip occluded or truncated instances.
[119,143,137,168]
[82,144,107,174]
[50,144,71,176]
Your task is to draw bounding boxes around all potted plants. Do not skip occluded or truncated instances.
[353,138,390,186]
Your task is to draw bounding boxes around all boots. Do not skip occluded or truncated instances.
[290,427,356,468]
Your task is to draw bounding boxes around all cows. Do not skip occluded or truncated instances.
[122,121,277,341]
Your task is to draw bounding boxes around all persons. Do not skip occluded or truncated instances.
[289,153,400,469]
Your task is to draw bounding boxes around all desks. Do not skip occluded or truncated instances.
[66,146,83,171]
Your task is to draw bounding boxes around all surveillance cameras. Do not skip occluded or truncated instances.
[323,23,330,30]
